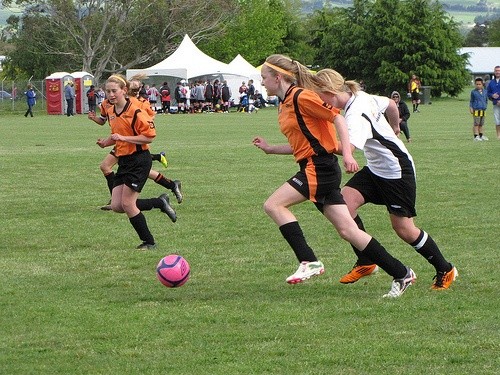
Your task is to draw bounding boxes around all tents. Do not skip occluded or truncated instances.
[127,33,276,109]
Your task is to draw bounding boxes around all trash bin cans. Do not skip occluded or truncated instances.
[419,86,433,104]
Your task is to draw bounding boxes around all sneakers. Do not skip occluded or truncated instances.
[100,199,111,210]
[285,259,325,284]
[382,268,417,299]
[159,194,177,223]
[427,264,459,292]
[171,180,183,204]
[135,241,157,250]
[339,261,378,284]
[159,152,168,168]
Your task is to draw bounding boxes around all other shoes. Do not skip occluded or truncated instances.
[473,135,488,141]
[408,138,411,143]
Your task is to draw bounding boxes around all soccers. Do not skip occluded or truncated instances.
[156,255,191,289]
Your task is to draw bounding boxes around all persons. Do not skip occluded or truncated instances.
[65,81,76,116]
[390,91,411,142]
[408,75,421,113]
[89,74,183,249]
[469,78,488,141]
[313,70,458,290]
[96,87,105,109]
[87,85,98,113]
[24,85,35,117]
[176,79,231,113]
[237,80,267,112]
[487,66,500,140]
[253,54,417,299]
